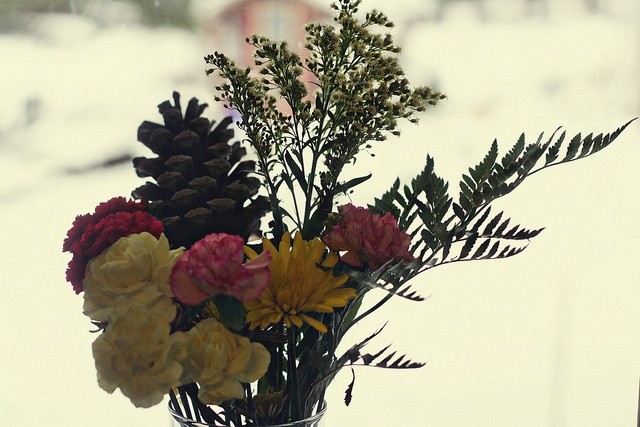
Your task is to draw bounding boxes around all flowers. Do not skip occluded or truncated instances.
[62,0,639,416]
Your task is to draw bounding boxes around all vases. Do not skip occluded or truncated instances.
[165,395,329,426]
